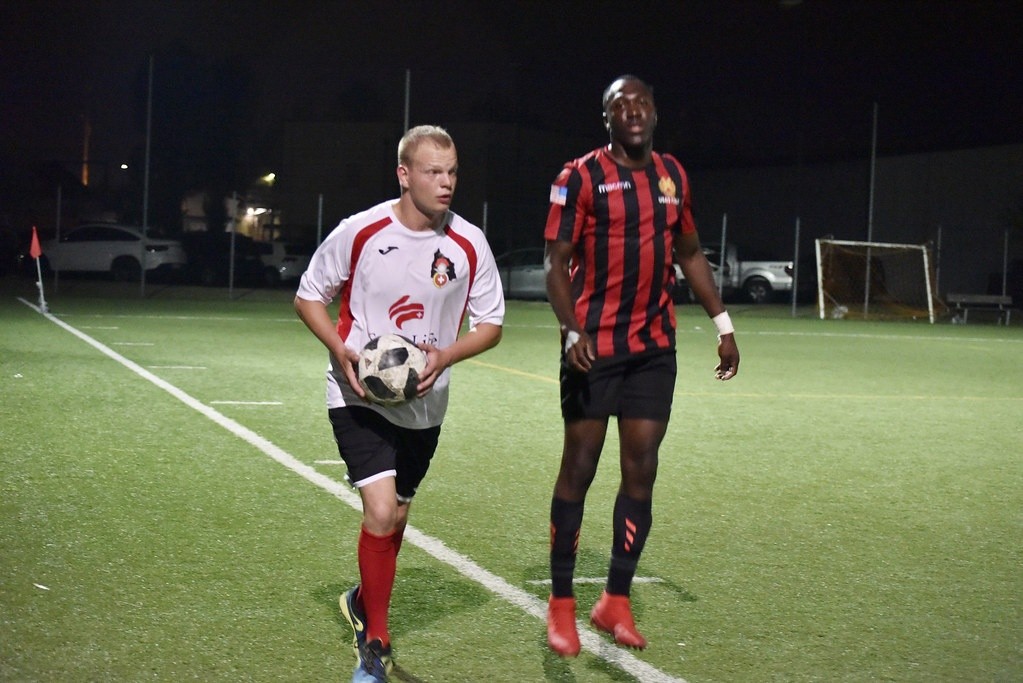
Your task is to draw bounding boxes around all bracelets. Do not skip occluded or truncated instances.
[712,311,734,335]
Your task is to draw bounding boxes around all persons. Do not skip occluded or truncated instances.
[542,74,740,656]
[294,125,505,683]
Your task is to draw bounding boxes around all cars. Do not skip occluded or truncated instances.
[493,247,582,301]
[16,220,189,283]
[671,246,731,307]
[232,240,314,288]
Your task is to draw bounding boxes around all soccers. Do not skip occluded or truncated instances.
[358,332,428,409]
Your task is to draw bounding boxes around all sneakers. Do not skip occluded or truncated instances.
[589,593,645,649]
[546,593,581,658]
[339,586,369,639]
[353,637,393,683]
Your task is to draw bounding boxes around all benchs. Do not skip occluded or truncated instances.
[945,293,1017,326]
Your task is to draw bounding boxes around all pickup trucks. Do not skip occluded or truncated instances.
[699,240,795,304]
